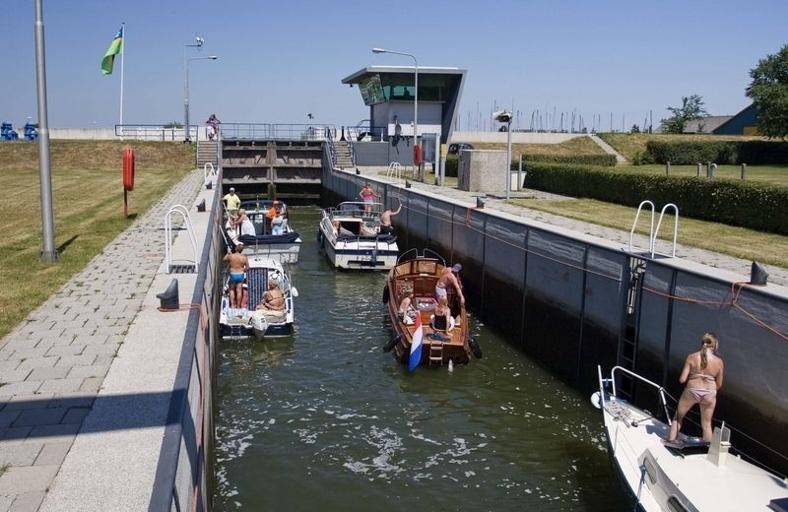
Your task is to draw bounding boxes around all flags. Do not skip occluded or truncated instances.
[99,26,123,75]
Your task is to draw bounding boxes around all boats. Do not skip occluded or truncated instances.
[317,195,402,275]
[232,196,302,266]
[217,253,300,342]
[381,245,473,367]
[588,361,788,510]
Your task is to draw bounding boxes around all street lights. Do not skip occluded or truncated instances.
[371,46,418,182]
[497,112,513,199]
[180,35,218,146]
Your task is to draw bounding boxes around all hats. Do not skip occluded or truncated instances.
[452,263,462,272]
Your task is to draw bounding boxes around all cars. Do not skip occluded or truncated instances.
[447,142,475,157]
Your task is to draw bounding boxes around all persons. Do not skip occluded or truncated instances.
[225,278,249,310]
[359,184,380,215]
[430,295,453,337]
[379,203,402,235]
[221,243,248,308]
[220,187,287,237]
[206,114,221,141]
[401,86,409,95]
[255,279,286,312]
[434,264,465,306]
[397,292,418,323]
[668,333,724,445]
